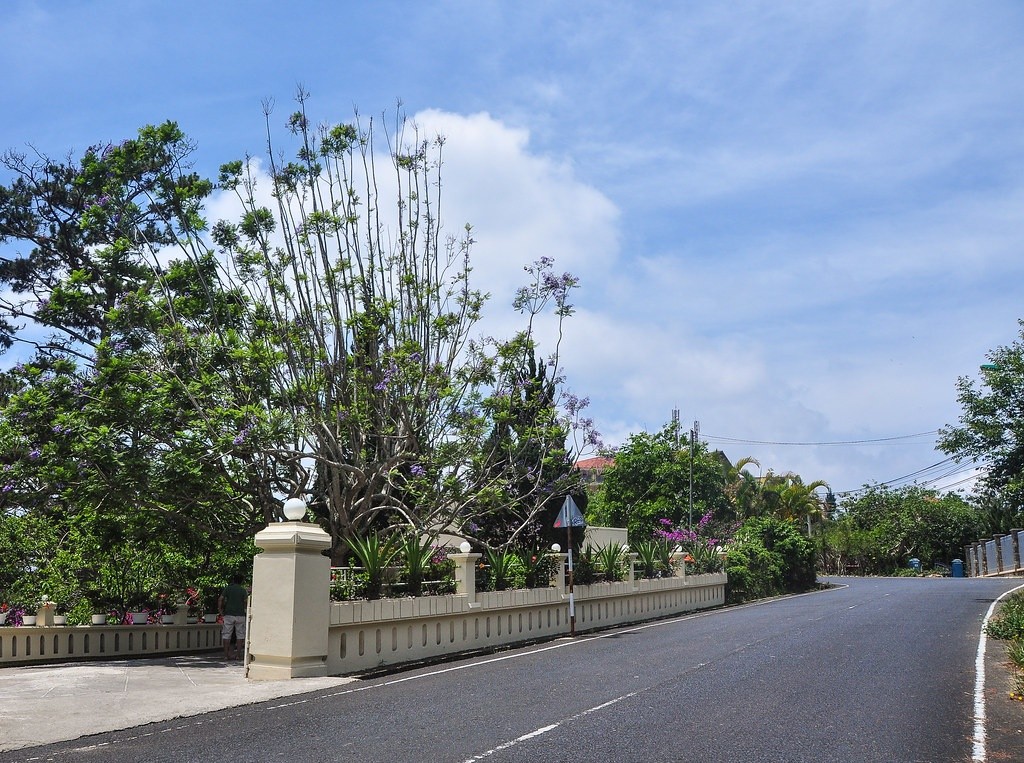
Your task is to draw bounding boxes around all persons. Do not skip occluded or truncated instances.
[218,574,252,660]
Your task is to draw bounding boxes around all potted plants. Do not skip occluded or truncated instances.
[129,593,148,624]
[187,606,200,624]
[161,597,177,624]
[54,603,69,625]
[21,606,38,625]
[87,590,107,625]
[203,598,218,623]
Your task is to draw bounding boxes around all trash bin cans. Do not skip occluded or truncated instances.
[951,559,963,577]
[909,558,921,569]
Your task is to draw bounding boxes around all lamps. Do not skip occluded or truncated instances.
[551,543,562,553]
[283,498,306,522]
[460,542,470,553]
[622,545,630,553]
[676,545,682,552]
[716,545,722,552]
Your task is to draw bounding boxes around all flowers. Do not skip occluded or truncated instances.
[0,604,26,627]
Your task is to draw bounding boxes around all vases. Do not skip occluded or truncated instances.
[0,614,5,624]
